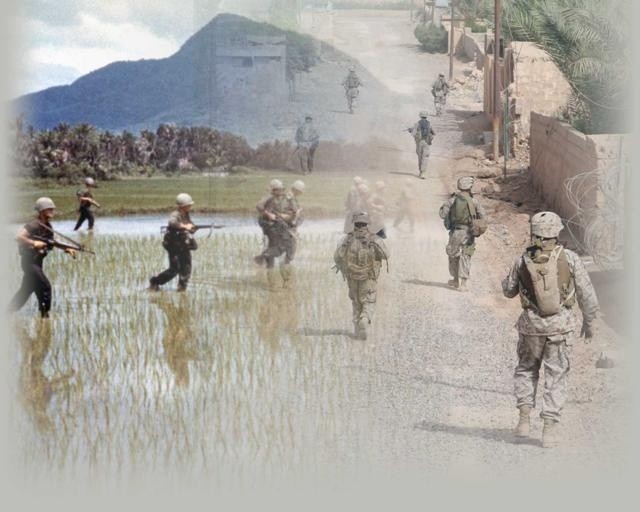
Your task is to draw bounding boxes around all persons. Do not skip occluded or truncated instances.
[294,113,319,176]
[333,210,391,340]
[73,177,99,231]
[254,178,306,290]
[501,212,600,450]
[431,71,450,116]
[438,175,489,291]
[4,196,77,318]
[341,175,419,239]
[148,192,199,291]
[409,110,436,180]
[341,65,364,114]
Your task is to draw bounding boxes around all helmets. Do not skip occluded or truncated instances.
[270,179,304,194]
[85,178,94,185]
[531,211,564,238]
[176,193,194,207]
[458,176,473,190]
[35,197,56,212]
[419,111,427,118]
[352,211,369,224]
[305,112,312,120]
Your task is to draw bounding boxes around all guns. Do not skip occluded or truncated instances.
[32,236,91,254]
[265,202,302,245]
[77,191,100,210]
[162,223,222,234]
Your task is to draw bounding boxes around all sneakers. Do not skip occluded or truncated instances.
[513,413,532,437]
[150,276,158,290]
[541,425,562,447]
[303,169,312,176]
[419,170,426,178]
[353,317,369,339]
[448,276,468,291]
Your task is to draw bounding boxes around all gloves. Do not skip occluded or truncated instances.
[579,319,594,344]
[65,247,77,257]
[34,240,47,249]
[186,223,194,230]
